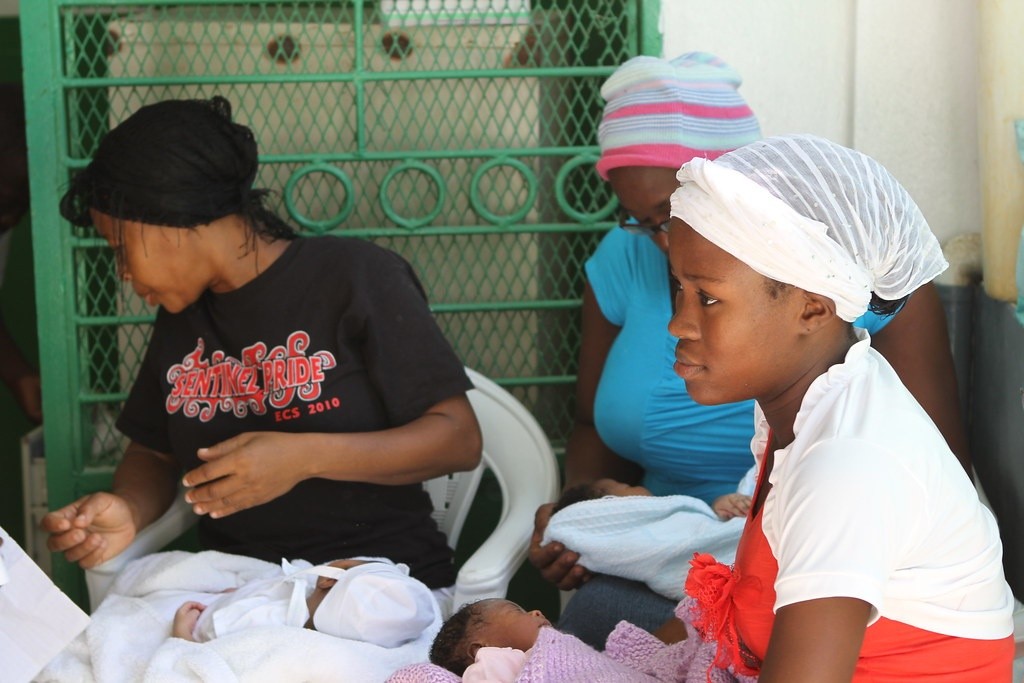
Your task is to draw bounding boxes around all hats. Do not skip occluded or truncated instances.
[312,561,436,649]
[594,51,766,182]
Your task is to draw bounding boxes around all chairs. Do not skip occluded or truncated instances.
[83,365,561,616]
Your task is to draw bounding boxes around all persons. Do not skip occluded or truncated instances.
[429,53,1015,683]
[42,99,482,648]
